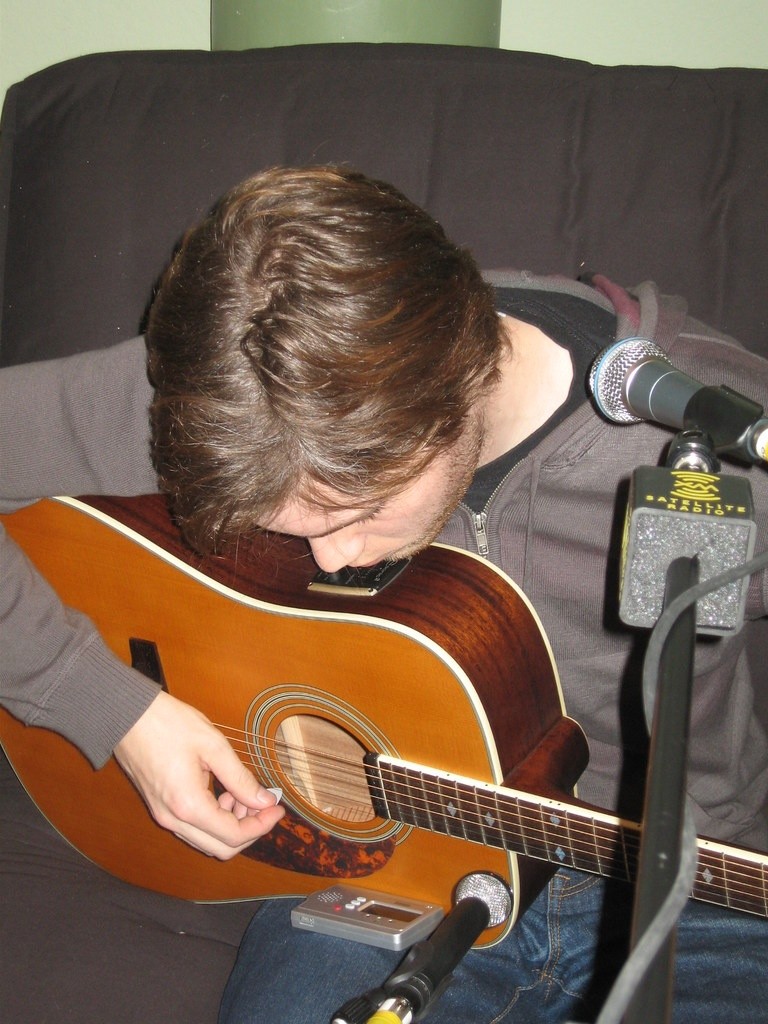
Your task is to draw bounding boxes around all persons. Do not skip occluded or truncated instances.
[0,172,768,1024]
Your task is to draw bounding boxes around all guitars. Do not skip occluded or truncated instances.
[0,486,768,949]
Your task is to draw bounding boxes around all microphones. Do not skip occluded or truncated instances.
[368,871,515,1024]
[589,336,768,465]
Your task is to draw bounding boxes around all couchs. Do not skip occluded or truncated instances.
[0,45,768,1024]
